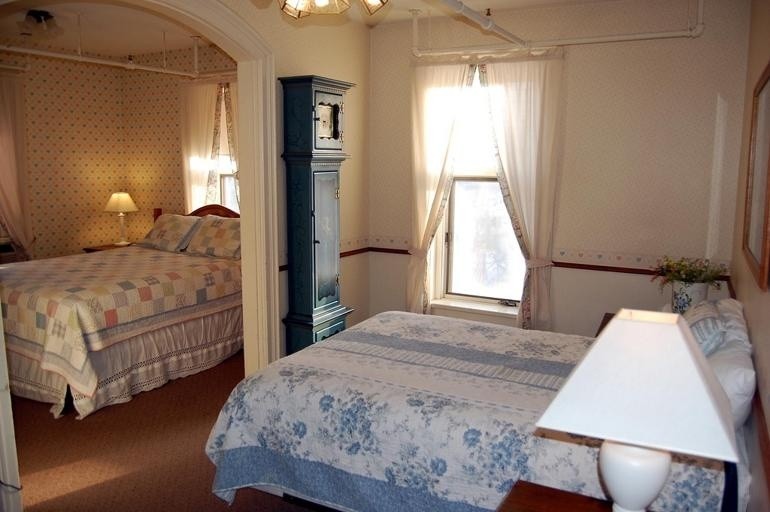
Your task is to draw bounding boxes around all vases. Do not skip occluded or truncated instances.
[672,280,708,313]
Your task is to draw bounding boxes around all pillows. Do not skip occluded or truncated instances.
[682,300,756,431]
[137,214,206,253]
[184,214,241,261]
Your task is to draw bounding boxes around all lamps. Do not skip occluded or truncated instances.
[17,9,64,40]
[103,192,139,246]
[277,0,389,21]
[534,309,739,512]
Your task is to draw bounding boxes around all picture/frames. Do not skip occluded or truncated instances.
[740,62,770,292]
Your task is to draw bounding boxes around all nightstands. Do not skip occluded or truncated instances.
[493,480,650,512]
[594,312,616,337]
[83,244,130,252]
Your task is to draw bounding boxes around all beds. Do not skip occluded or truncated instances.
[206,310,752,512]
[0,205,243,420]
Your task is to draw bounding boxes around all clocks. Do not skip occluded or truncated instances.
[277,75,360,357]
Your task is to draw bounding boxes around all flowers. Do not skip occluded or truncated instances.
[650,255,731,295]
